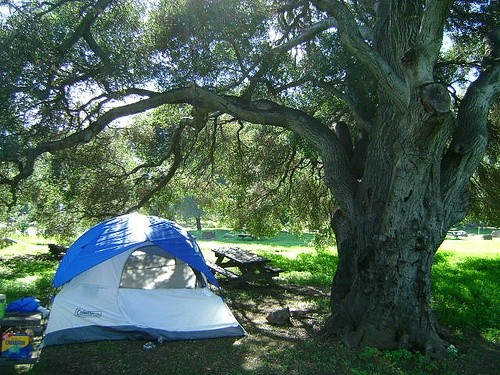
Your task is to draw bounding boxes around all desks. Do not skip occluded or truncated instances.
[211,247,273,288]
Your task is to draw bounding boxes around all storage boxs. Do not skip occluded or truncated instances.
[1,327,34,358]
[0,293,6,320]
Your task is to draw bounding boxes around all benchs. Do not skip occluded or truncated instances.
[263,263,285,277]
[448,231,468,238]
[204,259,244,287]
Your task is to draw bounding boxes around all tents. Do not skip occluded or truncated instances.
[40,210,248,348]
